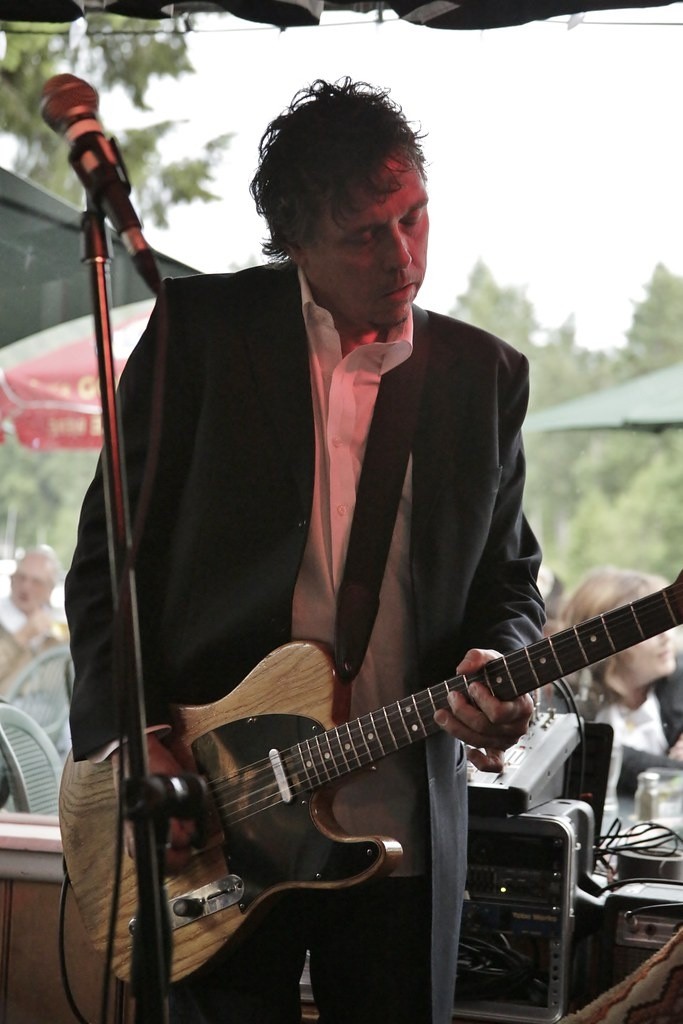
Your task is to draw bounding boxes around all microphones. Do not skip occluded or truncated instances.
[36,73,163,294]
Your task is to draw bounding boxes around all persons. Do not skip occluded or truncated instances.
[534,564,683,798]
[60,79,545,1022]
[0,545,68,694]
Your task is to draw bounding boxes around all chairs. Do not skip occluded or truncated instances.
[5,645,74,744]
[0,703,62,815]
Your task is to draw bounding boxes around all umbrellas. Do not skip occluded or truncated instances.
[1,300,160,452]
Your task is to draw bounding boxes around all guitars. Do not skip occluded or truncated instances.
[55,571,683,987]
